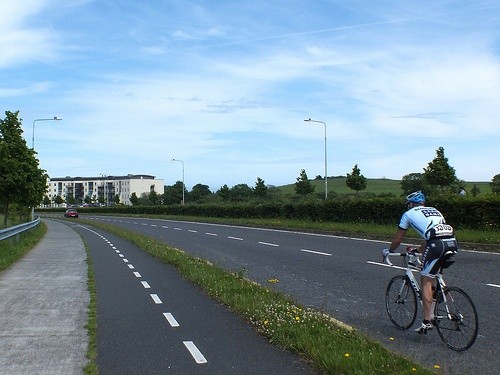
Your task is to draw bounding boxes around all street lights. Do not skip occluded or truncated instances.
[304,118,328,203]
[30,114,63,228]
[171,157,186,207]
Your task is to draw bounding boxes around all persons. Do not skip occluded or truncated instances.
[387,192,458,334]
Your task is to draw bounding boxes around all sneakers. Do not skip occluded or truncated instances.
[415,322,435,332]
[433,293,438,302]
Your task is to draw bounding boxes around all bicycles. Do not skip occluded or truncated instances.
[382,248,480,353]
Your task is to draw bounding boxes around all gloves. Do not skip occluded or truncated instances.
[382,249,394,257]
[410,248,420,253]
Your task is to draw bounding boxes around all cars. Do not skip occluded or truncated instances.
[63,208,80,219]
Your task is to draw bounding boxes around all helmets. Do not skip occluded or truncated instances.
[405,190,426,203]
